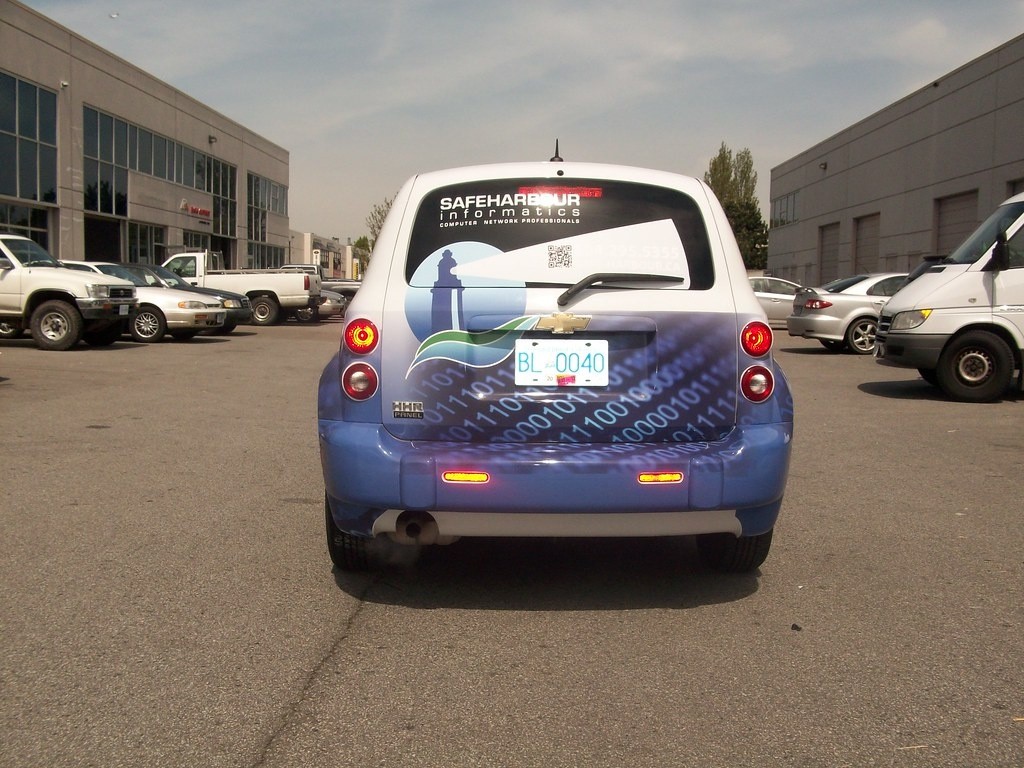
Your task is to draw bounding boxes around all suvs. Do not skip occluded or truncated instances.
[0,232,137,351]
[896,251,1024,294]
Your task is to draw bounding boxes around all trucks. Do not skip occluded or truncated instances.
[157,249,321,325]
[280,264,362,318]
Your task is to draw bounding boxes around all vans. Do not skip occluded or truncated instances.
[873,192,1024,403]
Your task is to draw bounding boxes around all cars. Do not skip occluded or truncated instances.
[316,159,795,573]
[0,258,227,342]
[295,290,346,323]
[748,277,802,325]
[788,273,911,354]
[113,263,253,335]
